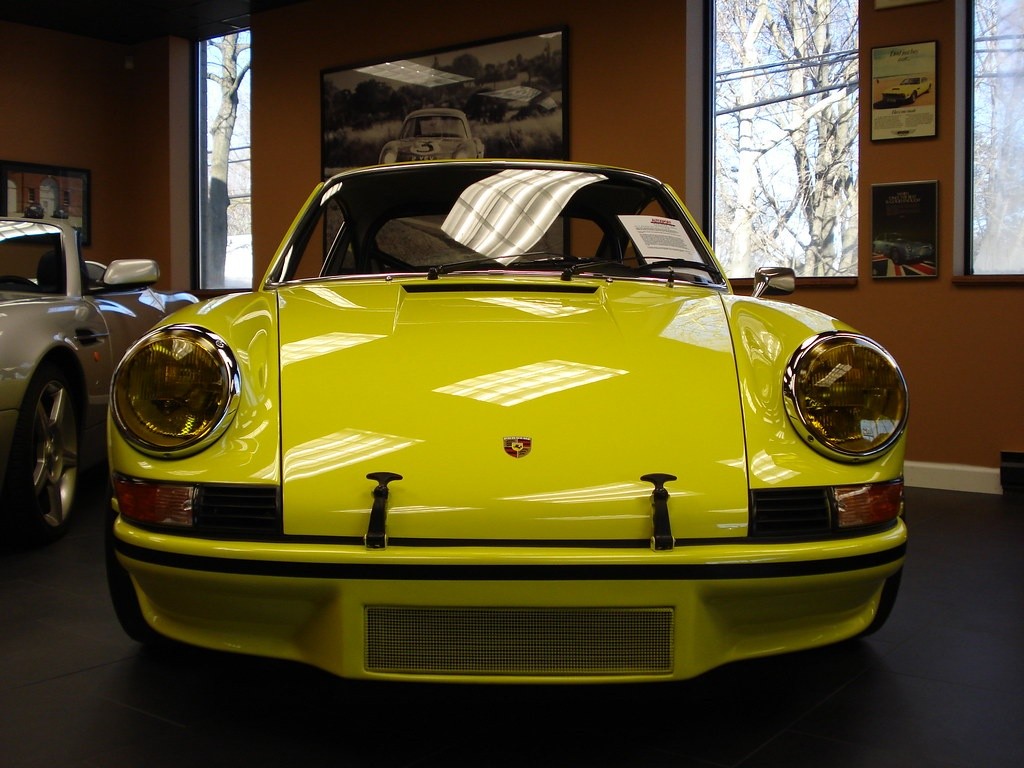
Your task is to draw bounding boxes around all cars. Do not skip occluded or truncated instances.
[881,75,932,106]
[110,155,907,716]
[1,215,202,543]
[379,109,485,164]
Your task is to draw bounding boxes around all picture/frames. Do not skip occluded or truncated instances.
[868,41,939,143]
[869,180,938,280]
[319,25,571,275]
[0,159,92,247]
